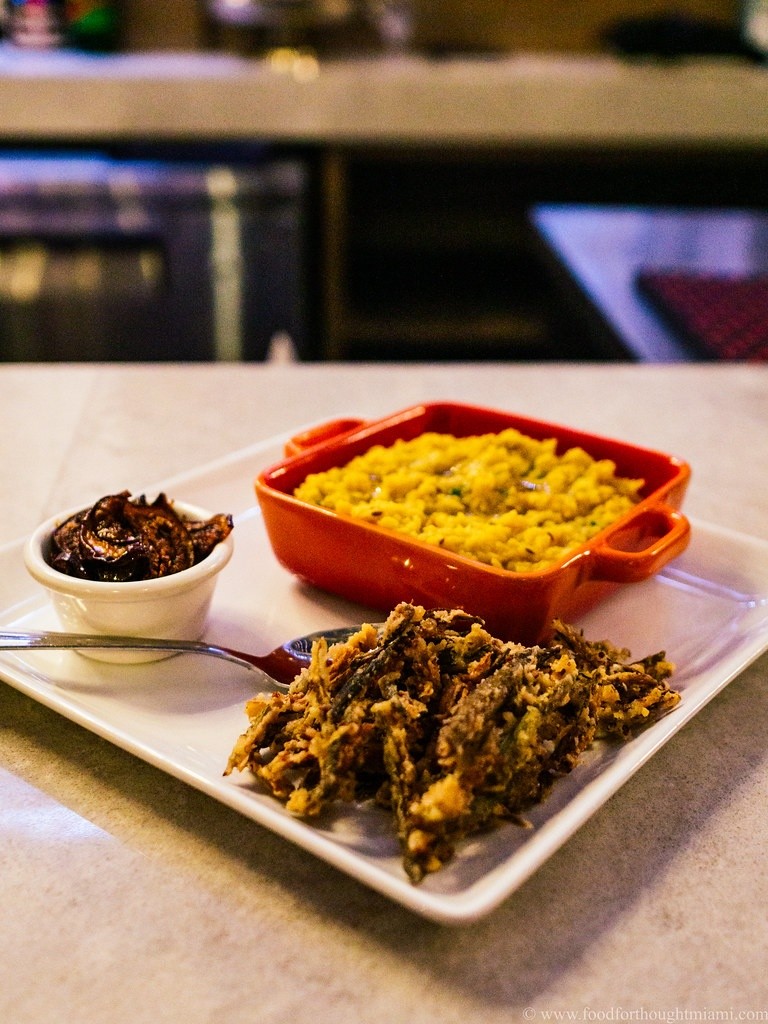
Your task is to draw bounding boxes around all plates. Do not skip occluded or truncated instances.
[0,413,768,926]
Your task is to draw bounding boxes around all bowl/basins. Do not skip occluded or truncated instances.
[255,400,692,646]
[24,499,234,665]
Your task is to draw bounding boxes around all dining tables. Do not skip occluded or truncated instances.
[0,361,768,1024]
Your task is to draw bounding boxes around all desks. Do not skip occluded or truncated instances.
[522,205,768,362]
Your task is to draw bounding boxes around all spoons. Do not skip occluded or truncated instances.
[0,626,364,689]
